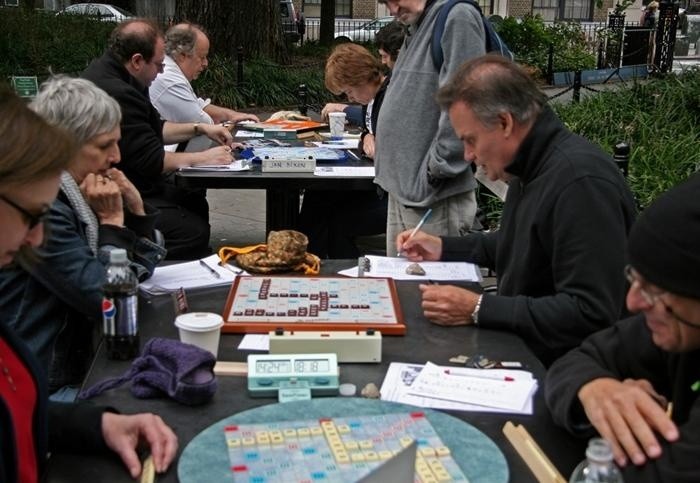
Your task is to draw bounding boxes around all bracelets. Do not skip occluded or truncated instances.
[192,122,201,135]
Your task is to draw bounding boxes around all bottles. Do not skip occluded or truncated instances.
[569,439,623,482]
[97,247,140,362]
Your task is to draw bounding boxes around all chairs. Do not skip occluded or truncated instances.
[477,135,629,295]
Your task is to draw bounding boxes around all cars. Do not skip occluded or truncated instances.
[57,2,139,26]
[334,15,396,46]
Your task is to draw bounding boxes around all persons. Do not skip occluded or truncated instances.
[398,57,643,371]
[146,24,260,153]
[85,21,233,269]
[641,0,659,28]
[300,0,489,257]
[543,191,699,483]
[2,79,179,483]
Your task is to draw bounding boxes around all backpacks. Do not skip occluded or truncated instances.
[429,1,516,79]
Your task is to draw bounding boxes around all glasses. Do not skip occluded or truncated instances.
[623,263,674,309]
[0,194,52,231]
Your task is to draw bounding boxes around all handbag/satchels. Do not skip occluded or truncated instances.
[218,228,322,277]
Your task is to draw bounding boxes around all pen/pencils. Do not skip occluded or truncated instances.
[217,262,242,274]
[445,369,515,381]
[227,149,239,153]
[200,261,219,278]
[397,209,433,257]
[347,149,360,160]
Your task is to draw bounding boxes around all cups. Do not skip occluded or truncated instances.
[327,112,347,135]
[173,312,225,361]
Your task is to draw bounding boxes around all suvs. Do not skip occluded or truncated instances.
[277,0,306,44]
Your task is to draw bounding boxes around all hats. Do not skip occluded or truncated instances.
[622,167,700,305]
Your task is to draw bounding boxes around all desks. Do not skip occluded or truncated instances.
[172,113,376,245]
[51,257,588,482]
